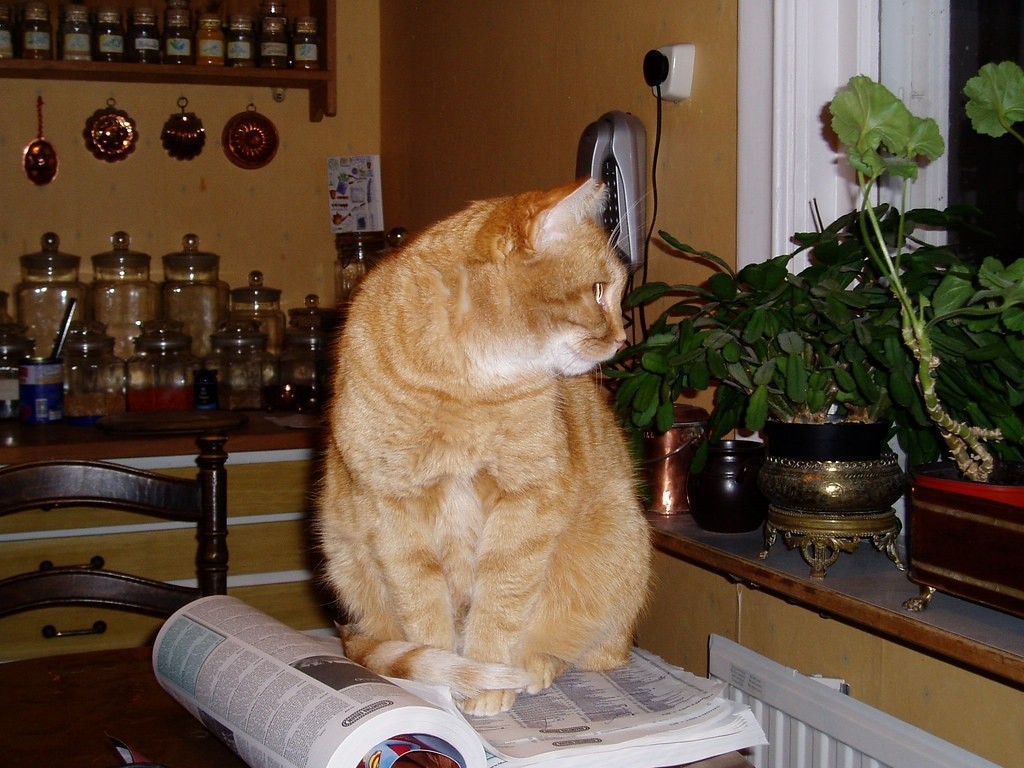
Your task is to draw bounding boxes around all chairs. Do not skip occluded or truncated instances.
[0,426,230,618]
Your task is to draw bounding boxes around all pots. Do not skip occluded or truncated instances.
[686,440,767,533]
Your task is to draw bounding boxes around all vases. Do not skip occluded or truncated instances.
[686,438,762,534]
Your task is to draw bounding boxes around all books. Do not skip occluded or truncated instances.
[152,595,769,768]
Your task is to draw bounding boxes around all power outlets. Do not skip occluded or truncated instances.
[653,43,696,102]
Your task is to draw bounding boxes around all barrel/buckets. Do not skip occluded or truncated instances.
[646,401,712,515]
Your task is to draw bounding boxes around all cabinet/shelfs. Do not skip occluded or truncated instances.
[0,447,354,662]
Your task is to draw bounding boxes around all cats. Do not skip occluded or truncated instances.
[318,179,654,715]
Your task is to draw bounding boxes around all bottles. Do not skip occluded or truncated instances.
[277,336,332,413]
[0,290,19,349]
[228,270,286,385]
[283,293,338,353]
[336,246,369,299]
[205,319,279,411]
[160,234,229,358]
[16,231,87,356]
[125,309,204,410]
[88,230,160,360]
[61,321,126,417]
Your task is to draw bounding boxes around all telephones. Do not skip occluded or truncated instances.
[574,110,648,273]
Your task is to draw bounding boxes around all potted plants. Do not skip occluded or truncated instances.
[587,60,1024,625]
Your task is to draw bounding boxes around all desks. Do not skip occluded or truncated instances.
[0,643,754,768]
